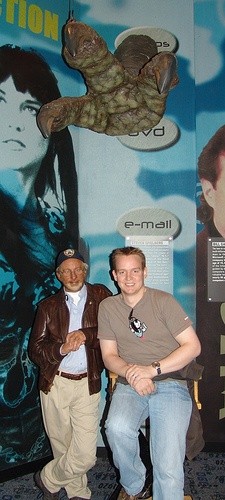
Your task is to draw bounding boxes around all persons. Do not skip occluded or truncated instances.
[186,125,225,500]
[0,45,79,466]
[28,248,112,500]
[96,247,201,500]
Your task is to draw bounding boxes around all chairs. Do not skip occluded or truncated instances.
[107,363,206,459]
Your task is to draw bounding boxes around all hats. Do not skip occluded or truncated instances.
[55,249,85,272]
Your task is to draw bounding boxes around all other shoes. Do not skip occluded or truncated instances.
[117,488,137,500]
[34,471,60,500]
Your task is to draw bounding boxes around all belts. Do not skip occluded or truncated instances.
[56,370,88,381]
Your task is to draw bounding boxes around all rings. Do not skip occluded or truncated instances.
[132,374,136,379]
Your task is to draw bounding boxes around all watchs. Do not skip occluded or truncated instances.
[151,361,161,377]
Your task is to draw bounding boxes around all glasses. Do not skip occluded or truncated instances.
[128,309,140,332]
